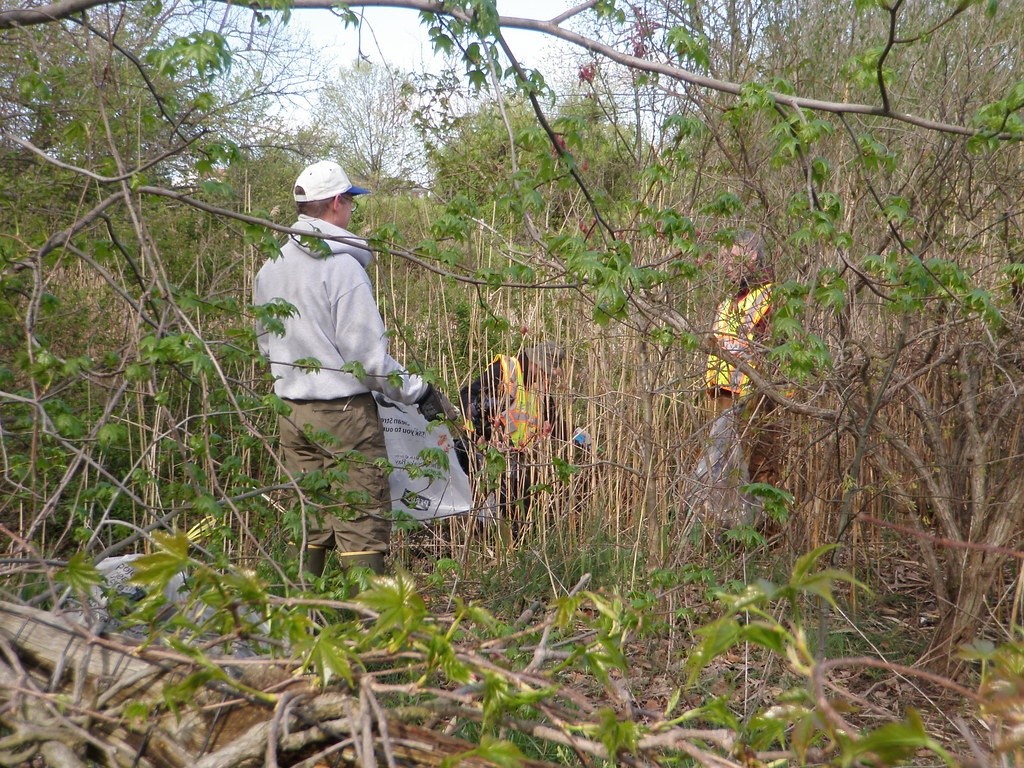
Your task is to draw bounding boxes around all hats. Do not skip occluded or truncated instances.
[525,339,565,378]
[293,161,371,202]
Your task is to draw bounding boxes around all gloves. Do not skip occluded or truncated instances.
[572,427,592,450]
[416,381,458,423]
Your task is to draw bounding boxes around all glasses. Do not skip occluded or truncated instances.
[341,195,359,212]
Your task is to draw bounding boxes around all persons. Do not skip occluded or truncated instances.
[704,228,789,554]
[457,337,593,545]
[251,158,459,634]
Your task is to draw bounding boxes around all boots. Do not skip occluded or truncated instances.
[338,548,388,631]
[287,540,326,635]
